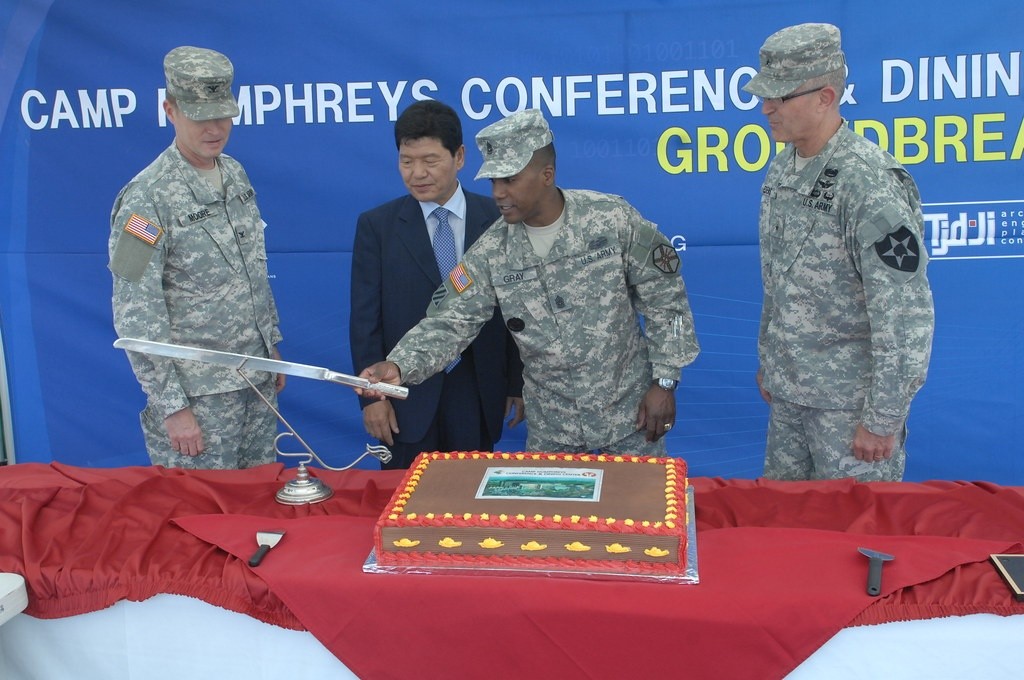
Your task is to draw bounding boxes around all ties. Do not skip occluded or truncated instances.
[432,207,461,374]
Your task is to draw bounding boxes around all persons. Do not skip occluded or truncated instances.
[742,22,935,482]
[356,108,701,461]
[107,46,285,469]
[349,100,524,471]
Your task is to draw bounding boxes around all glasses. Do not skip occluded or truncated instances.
[755,87,825,106]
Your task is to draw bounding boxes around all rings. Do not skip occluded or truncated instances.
[664,424,671,431]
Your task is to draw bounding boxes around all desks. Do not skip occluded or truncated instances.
[0,461,1024,680]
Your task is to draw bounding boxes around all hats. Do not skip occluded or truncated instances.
[742,23,846,99]
[474,108,555,181]
[163,46,240,121]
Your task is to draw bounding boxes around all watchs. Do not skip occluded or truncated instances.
[653,378,678,391]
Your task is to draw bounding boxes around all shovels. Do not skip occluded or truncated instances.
[248,527,288,567]
[857,546,896,596]
